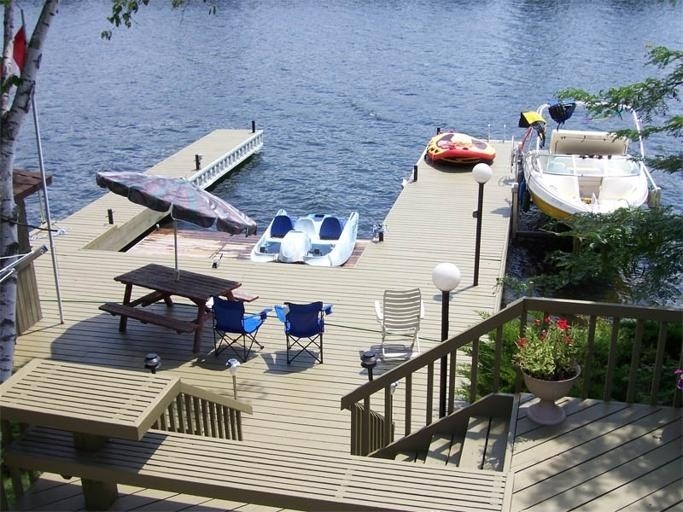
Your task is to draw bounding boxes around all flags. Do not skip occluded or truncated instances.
[0,9,34,93]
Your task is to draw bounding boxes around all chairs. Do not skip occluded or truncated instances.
[283,301,326,366]
[374,286,425,363]
[212,301,265,363]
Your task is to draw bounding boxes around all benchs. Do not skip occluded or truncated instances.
[98,301,196,334]
[216,282,261,303]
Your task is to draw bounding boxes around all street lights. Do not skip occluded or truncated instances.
[472,162,491,286]
[432,263,461,421]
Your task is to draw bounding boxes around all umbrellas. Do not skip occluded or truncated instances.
[96,171,257,283]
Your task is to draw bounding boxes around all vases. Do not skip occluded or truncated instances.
[521,356,580,427]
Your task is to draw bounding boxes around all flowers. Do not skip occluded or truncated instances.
[513,315,582,378]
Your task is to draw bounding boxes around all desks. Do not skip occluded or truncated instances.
[113,263,239,354]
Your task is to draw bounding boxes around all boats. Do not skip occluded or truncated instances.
[250,209,359,267]
[523,102,649,222]
